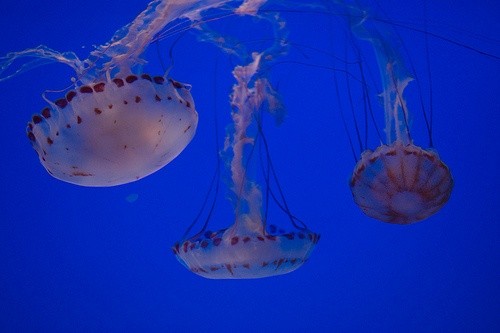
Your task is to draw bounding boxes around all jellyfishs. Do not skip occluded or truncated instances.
[171,0,322,279]
[0,0,235,186]
[333,8,453,227]
